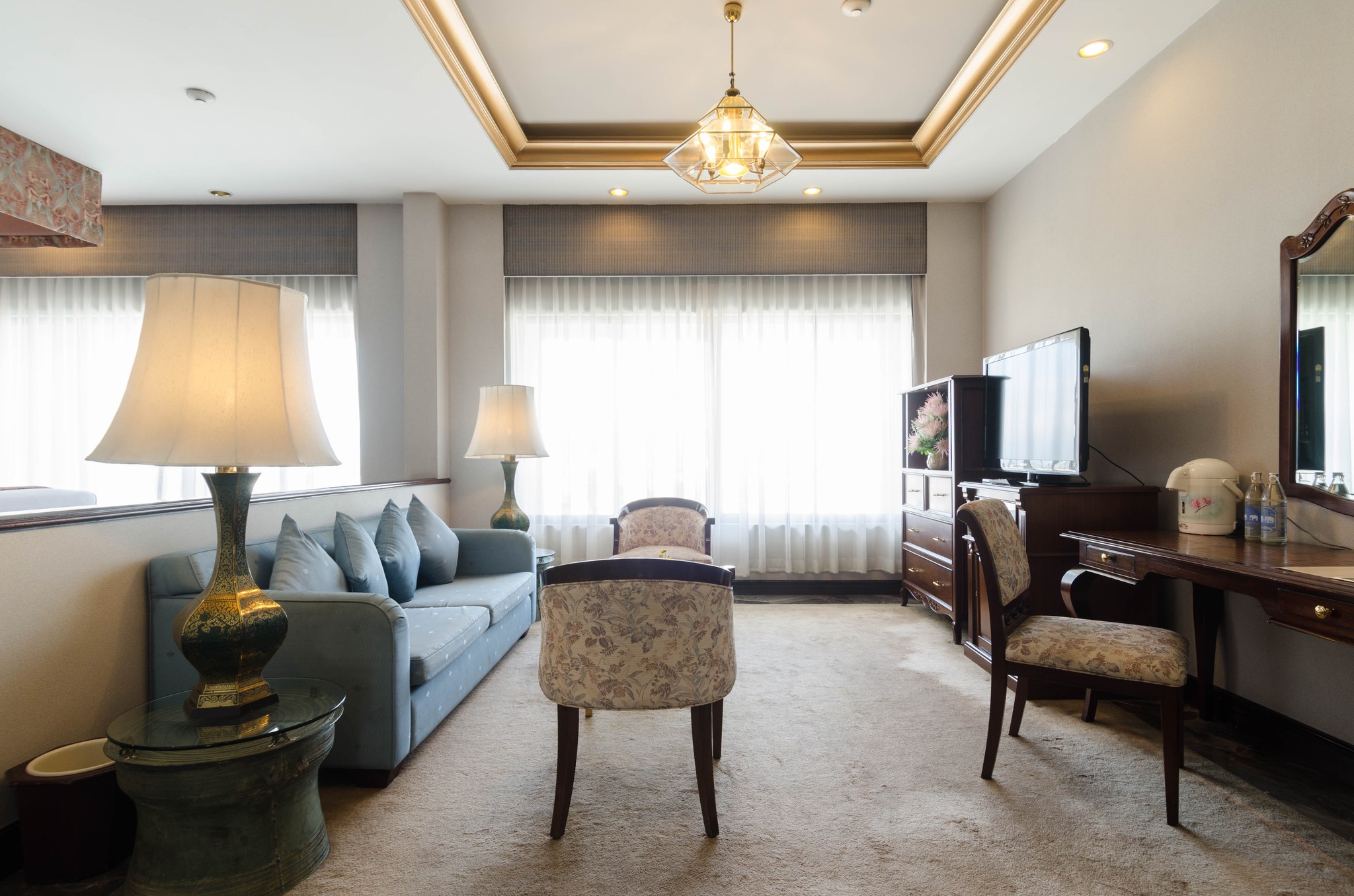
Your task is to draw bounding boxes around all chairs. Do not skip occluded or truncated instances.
[539,557,736,838]
[609,496,715,565]
[956,499,1188,829]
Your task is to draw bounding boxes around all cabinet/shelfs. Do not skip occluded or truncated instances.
[957,479,1161,700]
[898,375,982,644]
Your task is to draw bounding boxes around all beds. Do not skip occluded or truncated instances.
[0,485,97,513]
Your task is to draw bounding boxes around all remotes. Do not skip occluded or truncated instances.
[989,478,1017,487]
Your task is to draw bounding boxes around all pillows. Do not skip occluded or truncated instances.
[268,513,351,593]
[334,511,391,600]
[407,494,459,585]
[374,498,420,604]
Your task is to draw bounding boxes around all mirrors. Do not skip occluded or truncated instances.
[1280,186,1354,521]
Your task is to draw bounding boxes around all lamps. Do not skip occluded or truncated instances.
[660,0,803,196]
[85,270,342,722]
[462,384,551,532]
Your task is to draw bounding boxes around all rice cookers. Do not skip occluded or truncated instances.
[1165,458,1244,535]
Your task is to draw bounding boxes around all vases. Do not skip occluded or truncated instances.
[926,447,949,470]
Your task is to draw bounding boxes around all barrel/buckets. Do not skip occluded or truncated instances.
[4,735,136,885]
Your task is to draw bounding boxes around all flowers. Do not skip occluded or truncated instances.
[659,549,671,560]
[904,388,949,460]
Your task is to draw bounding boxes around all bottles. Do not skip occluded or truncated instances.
[1244,472,1265,541]
[1260,472,1287,543]
[1328,472,1351,496]
[1312,471,1327,487]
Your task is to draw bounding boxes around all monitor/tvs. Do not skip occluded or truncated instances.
[1298,326,1325,481]
[983,327,1092,488]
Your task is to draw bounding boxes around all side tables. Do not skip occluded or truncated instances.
[536,547,556,620]
[102,676,348,896]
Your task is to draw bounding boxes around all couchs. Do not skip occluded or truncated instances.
[144,506,539,788]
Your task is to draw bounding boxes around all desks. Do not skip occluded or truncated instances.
[1058,528,1354,723]
